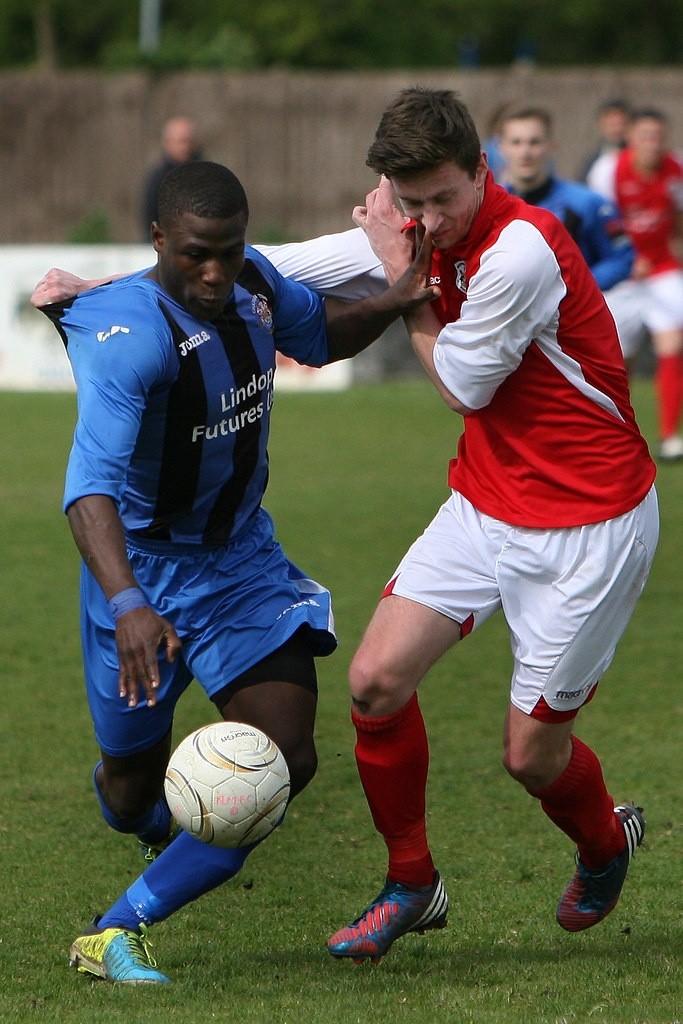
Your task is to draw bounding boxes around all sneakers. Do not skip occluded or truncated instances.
[67,914,172,987]
[137,811,182,864]
[325,869,450,963]
[555,803,647,933]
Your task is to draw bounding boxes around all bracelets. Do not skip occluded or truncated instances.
[107,586,148,624]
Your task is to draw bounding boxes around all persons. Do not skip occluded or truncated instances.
[137,90,683,464]
[319,90,661,966]
[34,161,443,990]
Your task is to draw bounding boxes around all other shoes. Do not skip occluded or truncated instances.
[659,438,682,463]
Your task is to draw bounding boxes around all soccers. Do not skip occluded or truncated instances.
[164,722,290,846]
